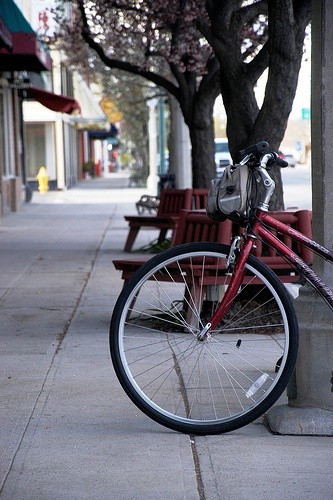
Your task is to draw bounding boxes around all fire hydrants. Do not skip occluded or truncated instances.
[35,166,50,193]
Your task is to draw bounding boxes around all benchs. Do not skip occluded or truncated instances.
[111,179,312,332]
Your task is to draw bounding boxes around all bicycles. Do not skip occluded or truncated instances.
[109,140,333,436]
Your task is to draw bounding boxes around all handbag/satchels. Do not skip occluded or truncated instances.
[206,154,252,224]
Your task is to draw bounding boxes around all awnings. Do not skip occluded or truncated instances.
[27,86,82,115]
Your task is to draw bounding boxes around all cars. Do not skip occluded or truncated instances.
[214,138,234,179]
[283,152,295,168]
